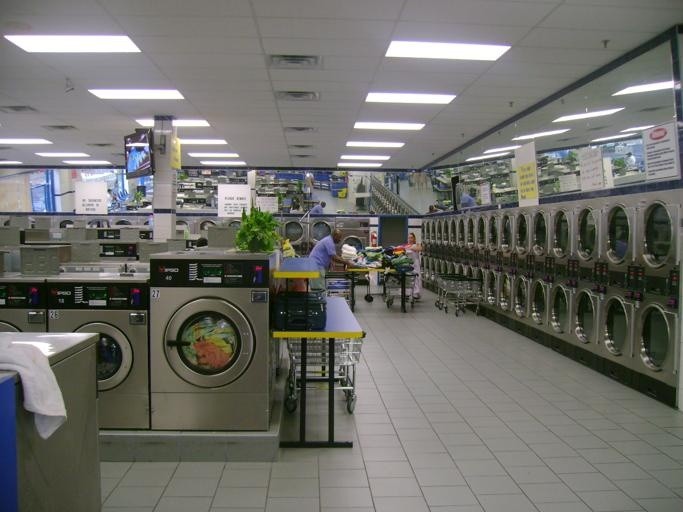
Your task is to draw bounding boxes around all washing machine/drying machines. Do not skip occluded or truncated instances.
[0,327,104,512]
[2,278,149,431]
[416,187,683,412]
[1,211,378,274]
[147,253,269,433]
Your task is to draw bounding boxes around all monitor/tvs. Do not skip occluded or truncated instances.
[124,129,154,179]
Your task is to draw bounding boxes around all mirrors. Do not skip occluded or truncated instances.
[420,21,682,218]
[0,166,420,216]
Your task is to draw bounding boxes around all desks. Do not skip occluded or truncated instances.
[347,268,407,313]
[273,259,365,448]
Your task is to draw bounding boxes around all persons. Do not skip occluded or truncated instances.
[405,233,423,302]
[289,171,326,214]
[461,187,479,208]
[107,189,117,207]
[308,229,355,297]
[624,152,635,168]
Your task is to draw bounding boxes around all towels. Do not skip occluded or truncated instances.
[1,339,73,445]
